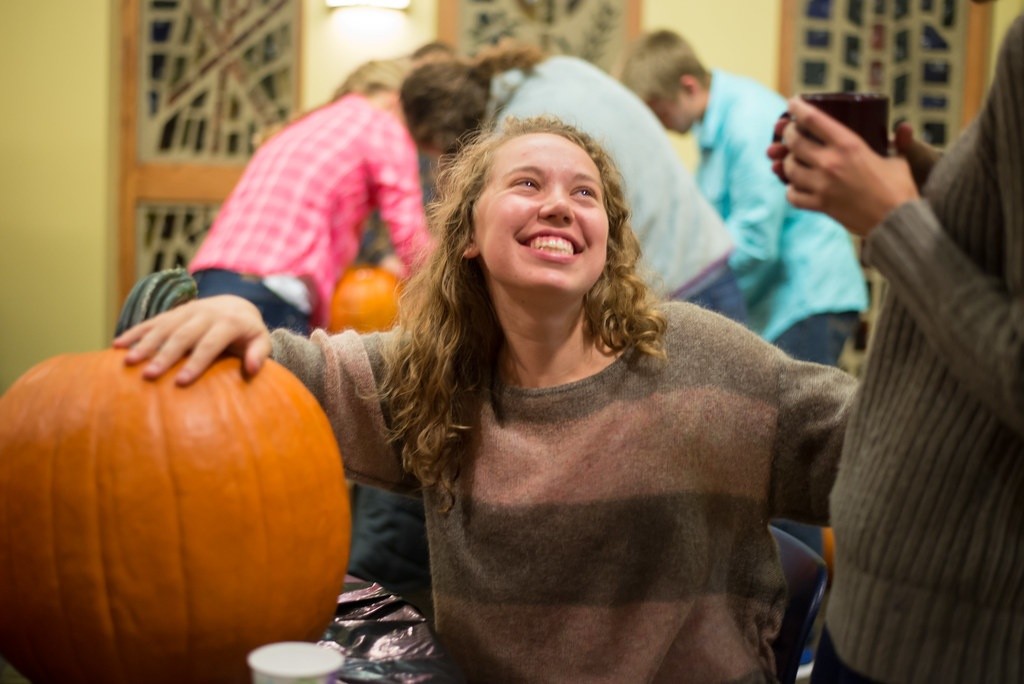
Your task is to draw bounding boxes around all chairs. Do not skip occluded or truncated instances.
[752,524,829,684]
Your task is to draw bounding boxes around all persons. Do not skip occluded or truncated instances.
[400,46,753,331]
[619,32,872,681]
[768,13,1024,684]
[111,117,861,684]
[176,65,483,409]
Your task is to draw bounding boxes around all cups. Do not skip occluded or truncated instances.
[246,641,345,684]
[772,93,888,185]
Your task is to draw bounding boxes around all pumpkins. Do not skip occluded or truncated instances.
[326,268,403,335]
[0,269,351,684]
